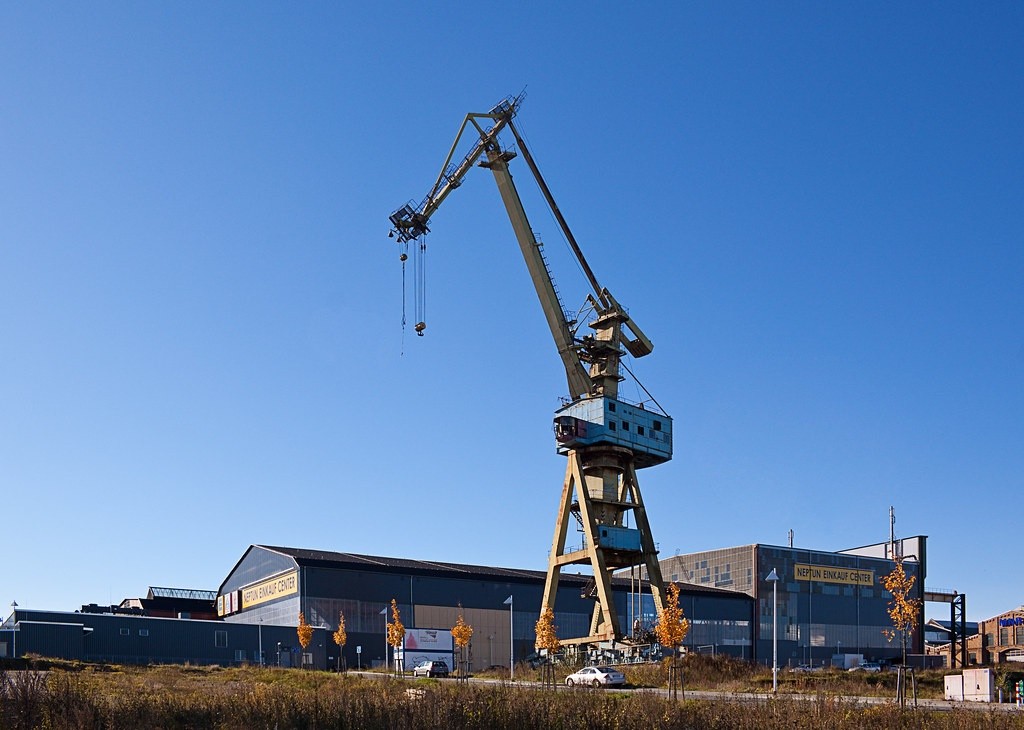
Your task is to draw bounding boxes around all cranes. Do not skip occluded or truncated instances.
[386,82,685,660]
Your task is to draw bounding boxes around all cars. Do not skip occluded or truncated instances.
[412,660,449,678]
[848,662,881,673]
[789,663,822,672]
[564,665,627,689]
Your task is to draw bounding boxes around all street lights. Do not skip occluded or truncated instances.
[766,567,780,696]
[803,643,807,664]
[379,607,389,673]
[504,595,515,680]
[837,640,841,655]
[258,616,264,669]
[10,600,20,659]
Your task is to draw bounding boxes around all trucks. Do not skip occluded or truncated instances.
[830,653,868,670]
